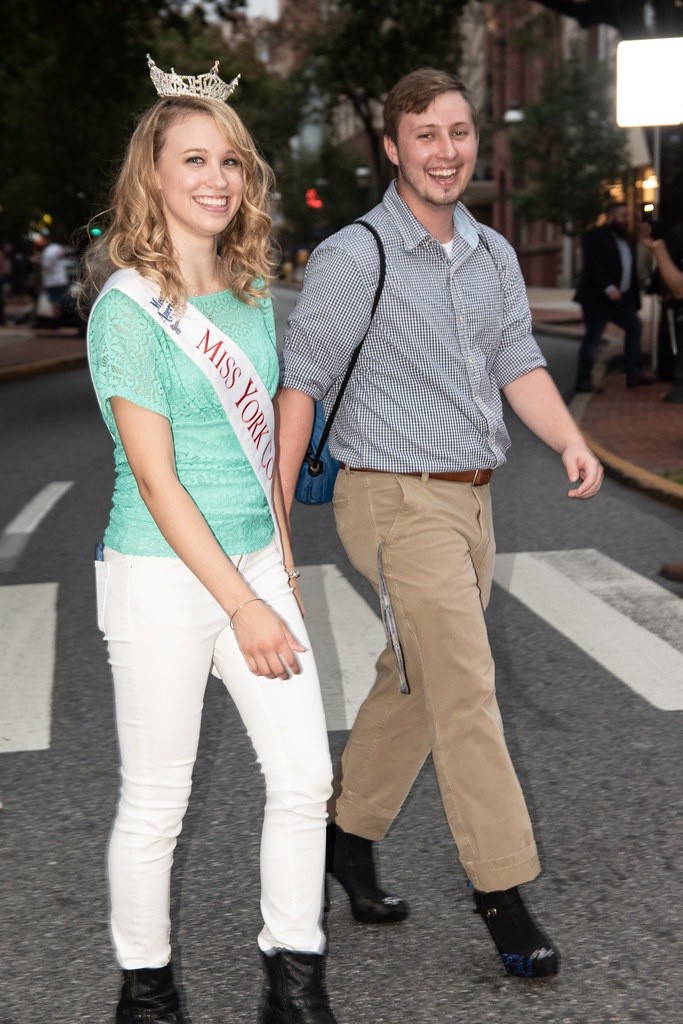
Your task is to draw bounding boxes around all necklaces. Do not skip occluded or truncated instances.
[175,260,220,320]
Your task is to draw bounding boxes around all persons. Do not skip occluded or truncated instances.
[69,96,339,1023]
[274,71,606,979]
[0,230,86,340]
[571,202,656,393]
[639,204,683,401]
[639,221,683,298]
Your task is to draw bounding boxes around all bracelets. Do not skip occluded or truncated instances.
[229,598,265,629]
[286,563,300,580]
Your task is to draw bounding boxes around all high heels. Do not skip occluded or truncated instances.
[472,886,560,978]
[325,820,408,924]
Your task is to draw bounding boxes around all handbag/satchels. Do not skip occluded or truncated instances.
[294,401,341,504]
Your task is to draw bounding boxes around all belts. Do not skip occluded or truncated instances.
[342,463,493,484]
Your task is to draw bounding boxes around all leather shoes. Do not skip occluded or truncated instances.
[115,966,181,1024]
[262,950,336,1024]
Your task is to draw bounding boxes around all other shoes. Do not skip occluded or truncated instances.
[575,387,603,393]
[625,376,656,387]
